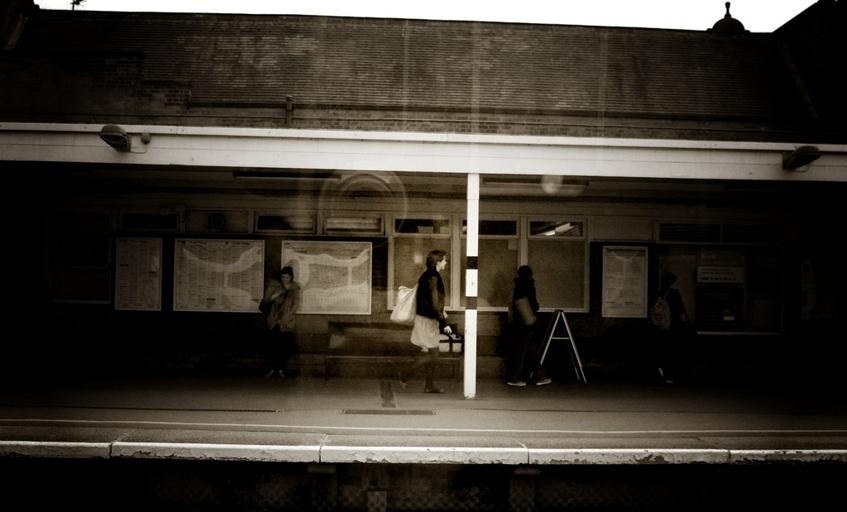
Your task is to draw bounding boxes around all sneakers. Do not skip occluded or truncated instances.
[507,377,551,387]
[263,370,286,379]
[424,385,445,393]
[398,369,408,391]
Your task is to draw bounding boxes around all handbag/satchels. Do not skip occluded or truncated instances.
[390,286,417,325]
[259,300,274,314]
[516,297,537,325]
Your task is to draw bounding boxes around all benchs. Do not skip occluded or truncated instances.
[291,355,462,388]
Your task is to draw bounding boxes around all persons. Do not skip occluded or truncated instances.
[504,265,553,387]
[648,269,689,389]
[396,250,453,395]
[261,265,302,380]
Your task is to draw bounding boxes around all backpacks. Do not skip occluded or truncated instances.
[651,288,671,328]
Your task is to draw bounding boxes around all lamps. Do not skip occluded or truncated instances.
[100,125,132,152]
[782,145,821,170]
